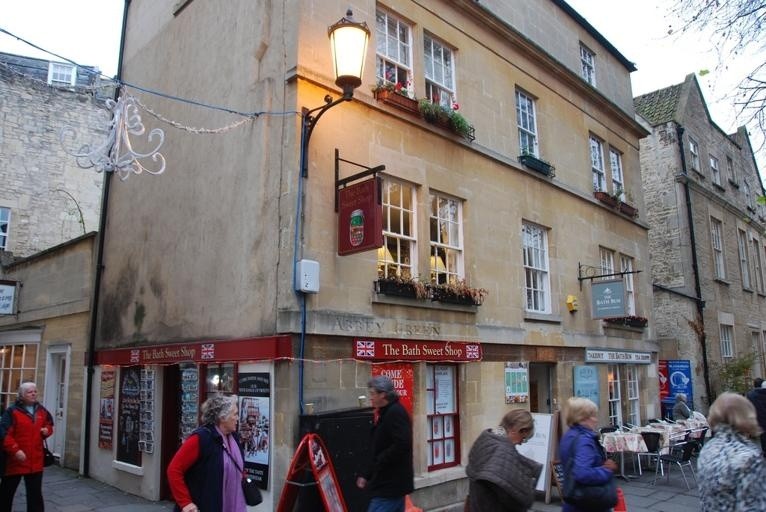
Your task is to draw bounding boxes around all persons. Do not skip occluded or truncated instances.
[672,392,692,421]
[463,408,543,512]
[165,395,247,512]
[695,392,766,512]
[354,376,414,512]
[559,397,619,512]
[0,382,53,512]
[745,377,766,457]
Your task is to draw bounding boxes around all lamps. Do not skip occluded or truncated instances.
[430,256,447,280]
[378,245,394,271]
[300,3,371,178]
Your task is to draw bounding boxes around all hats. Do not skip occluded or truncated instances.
[375,377,392,392]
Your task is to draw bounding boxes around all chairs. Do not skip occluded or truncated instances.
[598,419,710,491]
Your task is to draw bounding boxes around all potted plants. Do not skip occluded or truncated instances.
[375,270,488,305]
[522,144,551,176]
[594,186,639,217]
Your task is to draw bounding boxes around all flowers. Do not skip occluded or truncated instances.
[373,80,468,137]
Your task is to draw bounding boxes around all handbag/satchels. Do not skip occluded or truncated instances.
[563,474,618,509]
[241,478,263,506]
[43,447,55,466]
